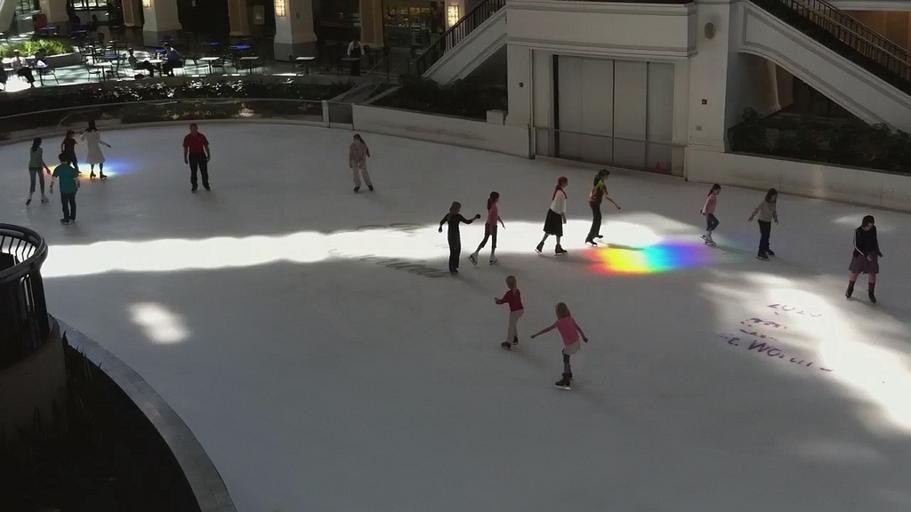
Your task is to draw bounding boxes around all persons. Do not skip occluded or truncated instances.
[182,124,211,192]
[162,43,182,76]
[529,302,588,388]
[0,49,46,83]
[471,191,505,264]
[25,120,111,222]
[537,176,568,253]
[127,48,153,77]
[494,275,524,349]
[585,168,621,245]
[349,134,374,193]
[749,188,779,259]
[846,215,882,303]
[700,183,721,243]
[439,201,481,272]
[347,37,365,76]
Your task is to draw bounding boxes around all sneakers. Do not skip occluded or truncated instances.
[868,290,876,302]
[501,336,572,386]
[846,287,853,297]
